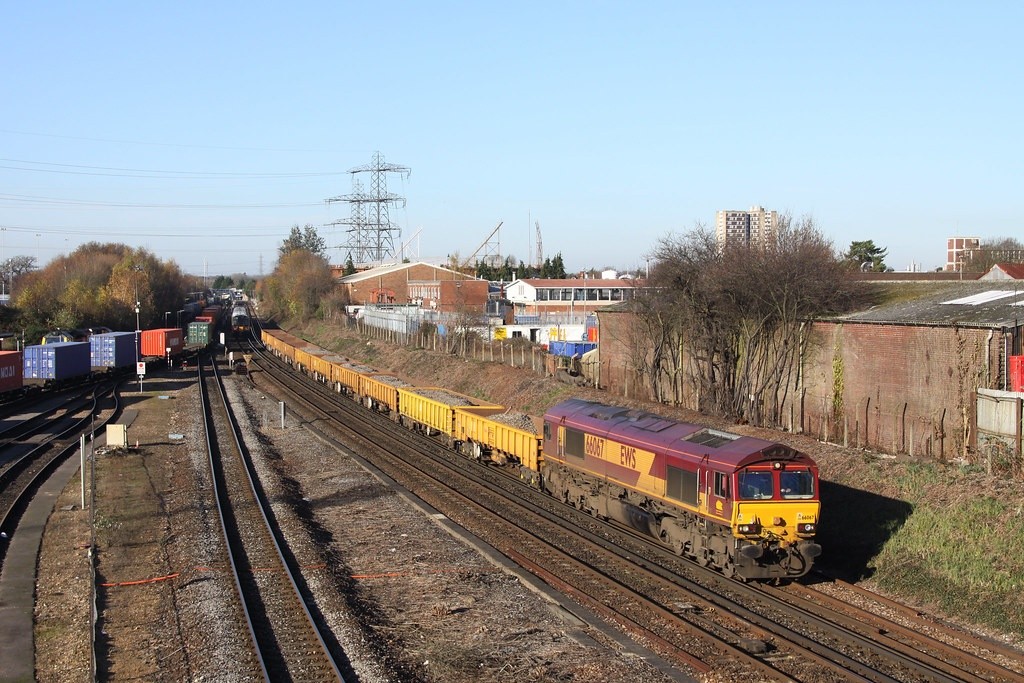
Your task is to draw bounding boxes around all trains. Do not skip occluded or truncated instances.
[232,307,250,334]
[542,399,823,584]
